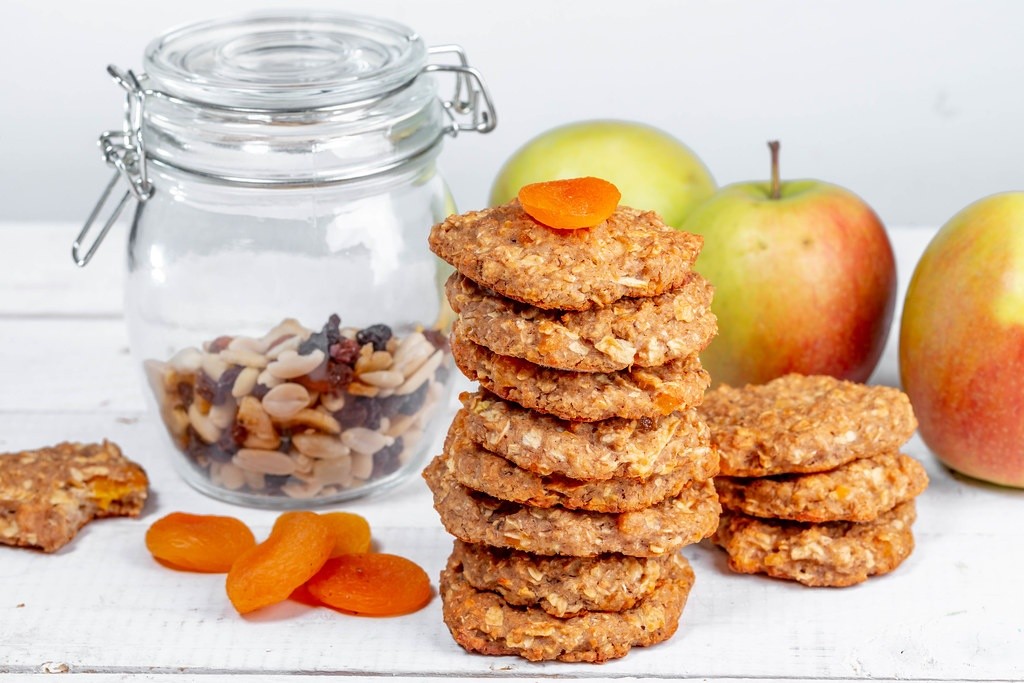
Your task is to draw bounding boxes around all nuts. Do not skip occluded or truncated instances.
[138,316,453,501]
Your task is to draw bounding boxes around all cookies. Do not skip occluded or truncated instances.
[0,442,146,553]
[424,178,929,664]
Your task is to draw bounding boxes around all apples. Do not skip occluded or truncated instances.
[488,118,1022,495]
